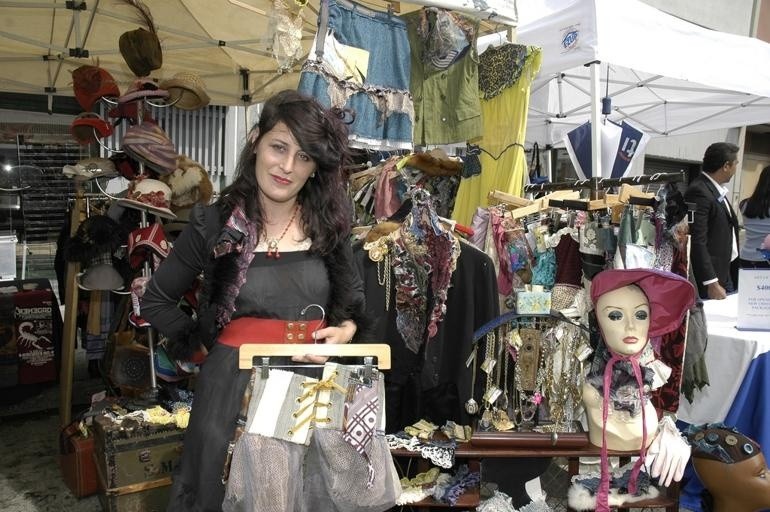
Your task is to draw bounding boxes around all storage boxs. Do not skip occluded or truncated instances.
[60,408,184,512]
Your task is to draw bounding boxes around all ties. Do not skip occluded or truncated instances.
[724,195,740,255]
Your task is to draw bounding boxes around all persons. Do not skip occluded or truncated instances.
[140,90,365,512]
[583,284,659,451]
[739,166,770,268]
[684,142,739,300]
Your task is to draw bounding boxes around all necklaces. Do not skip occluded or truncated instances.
[466,317,593,433]
[263,206,299,259]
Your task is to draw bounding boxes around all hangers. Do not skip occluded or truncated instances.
[405,189,430,242]
[487,172,698,224]
[347,149,401,214]
[238,304,392,371]
[493,22,510,49]
[357,0,395,15]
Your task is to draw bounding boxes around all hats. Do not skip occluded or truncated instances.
[70,113,115,147]
[122,121,177,175]
[72,64,120,113]
[117,80,169,104]
[63,153,212,328]
[590,268,694,338]
[119,27,163,78]
[159,73,211,112]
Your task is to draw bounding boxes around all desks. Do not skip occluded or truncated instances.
[389,429,679,511]
